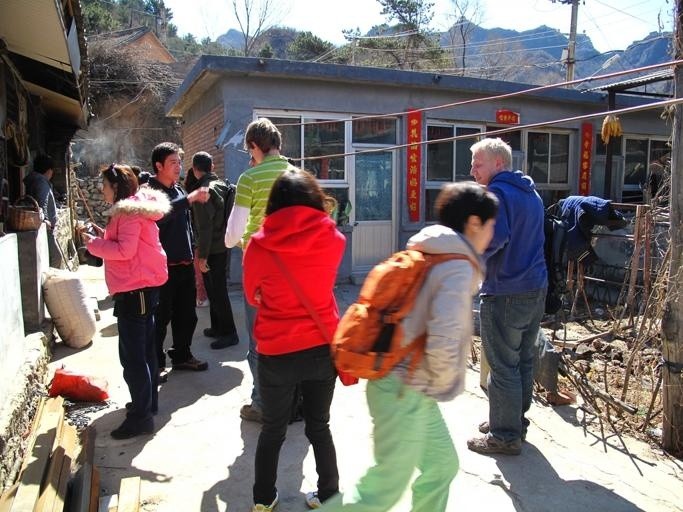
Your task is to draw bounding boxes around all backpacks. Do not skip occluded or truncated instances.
[330,249,481,385]
[224,178,236,238]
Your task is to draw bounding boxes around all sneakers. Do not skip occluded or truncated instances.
[467,434,522,456]
[251,491,279,512]
[210,333,239,349]
[173,354,208,370]
[204,328,221,338]
[240,405,264,422]
[127,402,158,415]
[306,491,323,508]
[110,416,154,440]
[480,421,527,442]
[157,369,168,382]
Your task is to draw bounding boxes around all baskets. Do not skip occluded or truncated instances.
[8,195,44,231]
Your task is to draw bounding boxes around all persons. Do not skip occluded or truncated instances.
[140,142,208,373]
[224,117,307,422]
[307,180,500,510]
[23,151,57,230]
[83,164,171,440]
[467,138,548,455]
[185,151,238,350]
[138,171,152,191]
[185,167,208,306]
[242,167,347,512]
